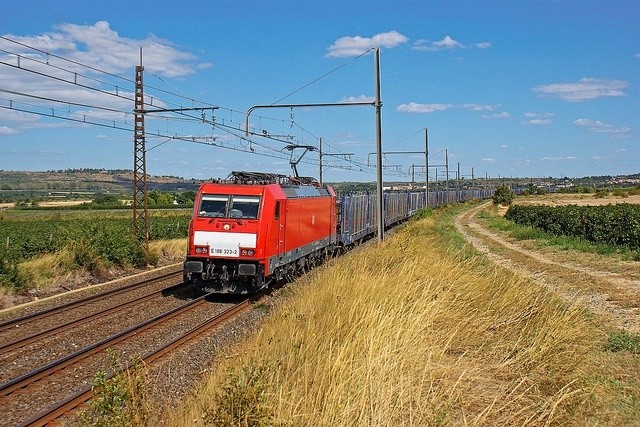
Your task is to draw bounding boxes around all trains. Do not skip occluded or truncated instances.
[183,171,555,303]
